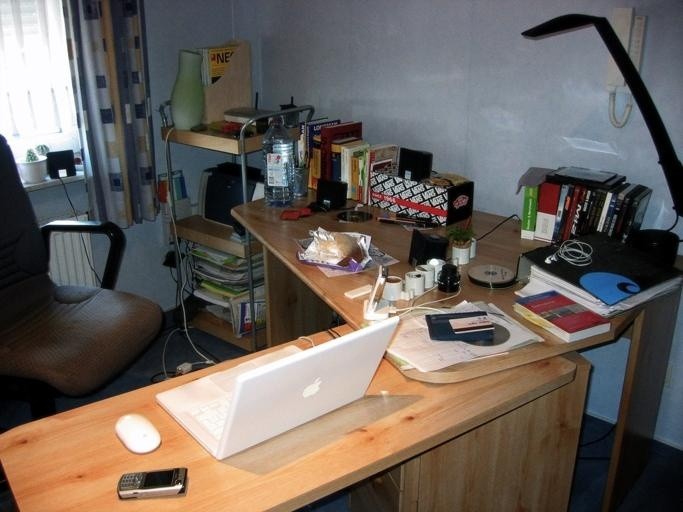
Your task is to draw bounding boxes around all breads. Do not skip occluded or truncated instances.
[319,235,360,258]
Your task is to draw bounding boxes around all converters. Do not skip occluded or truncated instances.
[176,363,193,374]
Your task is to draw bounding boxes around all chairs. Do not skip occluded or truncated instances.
[0,130,162,421]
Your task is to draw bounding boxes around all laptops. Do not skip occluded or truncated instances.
[155,315,402,460]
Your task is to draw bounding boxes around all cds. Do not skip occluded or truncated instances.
[337,210,373,222]
[463,324,510,346]
[468,263,515,288]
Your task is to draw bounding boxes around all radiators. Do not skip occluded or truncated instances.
[37,214,95,289]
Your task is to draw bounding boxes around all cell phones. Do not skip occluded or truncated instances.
[117,467,189,498]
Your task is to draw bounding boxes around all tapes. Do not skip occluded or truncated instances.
[383,256,448,299]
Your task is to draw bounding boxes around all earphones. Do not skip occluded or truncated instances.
[545,256,551,263]
[551,254,558,262]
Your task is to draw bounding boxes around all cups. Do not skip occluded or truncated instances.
[295,167,309,197]
[382,258,446,303]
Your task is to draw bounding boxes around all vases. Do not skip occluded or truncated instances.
[170,47,204,132]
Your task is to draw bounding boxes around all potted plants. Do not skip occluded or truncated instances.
[15,143,50,184]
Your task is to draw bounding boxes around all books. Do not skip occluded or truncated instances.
[220,105,279,126]
[197,44,239,88]
[514,163,655,250]
[291,114,398,208]
[188,230,267,339]
[512,288,612,344]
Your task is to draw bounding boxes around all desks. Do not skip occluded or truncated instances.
[0,180,683,512]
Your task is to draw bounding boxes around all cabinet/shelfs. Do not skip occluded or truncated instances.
[157,92,314,351]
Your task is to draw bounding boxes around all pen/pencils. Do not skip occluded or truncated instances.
[375,214,439,228]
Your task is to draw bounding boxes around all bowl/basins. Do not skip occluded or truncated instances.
[624,229,682,266]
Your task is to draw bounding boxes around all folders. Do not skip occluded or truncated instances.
[510,231,682,318]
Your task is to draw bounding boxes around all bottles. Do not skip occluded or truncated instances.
[437,264,459,293]
[262,116,295,203]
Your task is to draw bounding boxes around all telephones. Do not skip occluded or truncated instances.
[607,7,647,94]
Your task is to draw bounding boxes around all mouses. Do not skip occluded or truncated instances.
[114,413,162,455]
